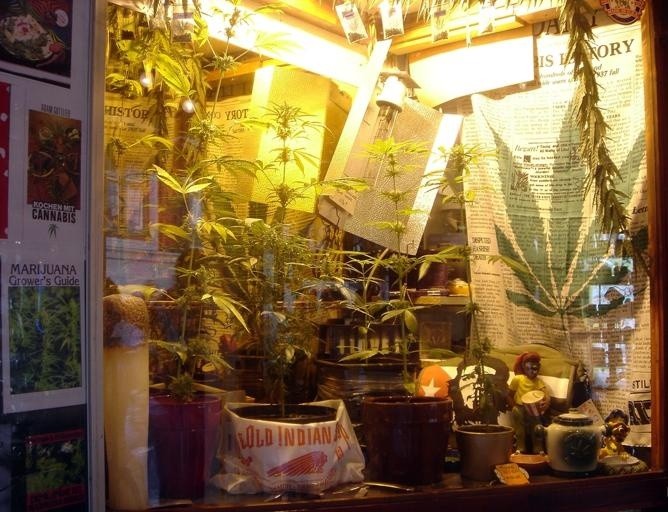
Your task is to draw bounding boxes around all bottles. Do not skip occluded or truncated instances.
[536,408,609,478]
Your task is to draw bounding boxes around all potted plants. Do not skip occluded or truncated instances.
[106,2,296,503]
[420,137,513,483]
[210,96,345,494]
[354,130,456,484]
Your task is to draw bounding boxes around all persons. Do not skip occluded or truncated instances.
[503,349,554,455]
